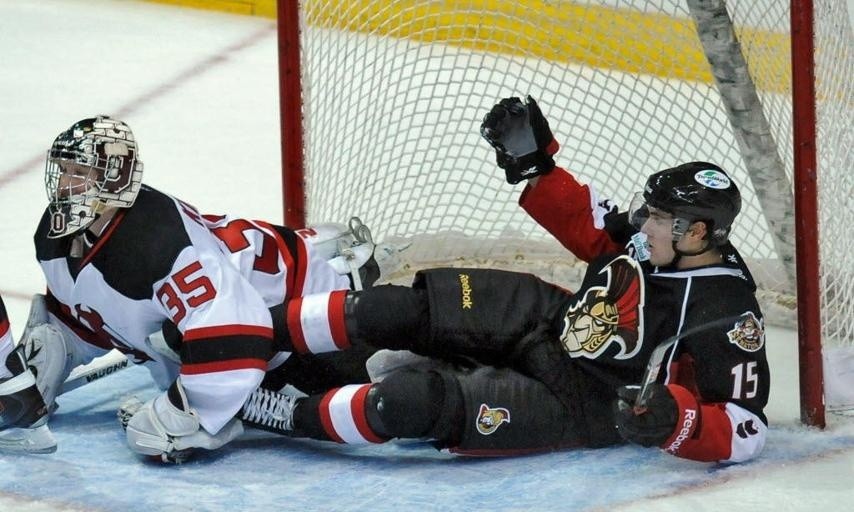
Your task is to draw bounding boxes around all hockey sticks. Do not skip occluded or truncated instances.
[635,316,749,408]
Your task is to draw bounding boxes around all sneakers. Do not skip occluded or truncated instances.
[237,388,303,440]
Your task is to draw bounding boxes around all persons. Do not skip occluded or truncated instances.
[117,91,770,468]
[0,110,381,469]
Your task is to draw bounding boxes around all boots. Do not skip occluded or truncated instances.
[1,345,49,431]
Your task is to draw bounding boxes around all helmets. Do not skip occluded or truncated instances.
[626,161,743,246]
[43,114,138,240]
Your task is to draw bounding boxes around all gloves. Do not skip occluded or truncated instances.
[613,385,677,448]
[480,94,561,183]
